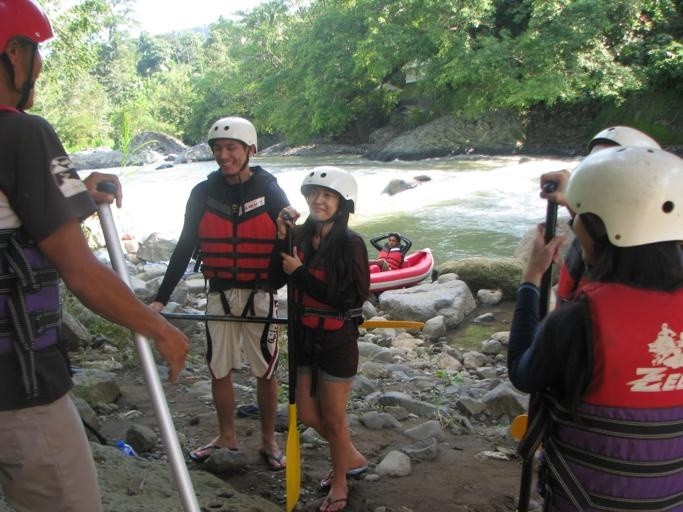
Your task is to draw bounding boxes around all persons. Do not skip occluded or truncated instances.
[148,116,291,471]
[0,0,190,512]
[554,125,663,308]
[368,232,412,272]
[505,142,683,512]
[269,165,371,512]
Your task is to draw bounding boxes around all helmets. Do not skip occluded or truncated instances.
[301,166,357,213]
[589,127,661,153]
[0,0,53,51]
[563,147,683,247]
[208,117,257,155]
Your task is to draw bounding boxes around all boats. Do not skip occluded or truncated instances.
[368,247,435,292]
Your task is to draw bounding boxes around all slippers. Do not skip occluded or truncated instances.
[189,443,239,462]
[317,485,350,512]
[320,462,369,490]
[259,447,286,472]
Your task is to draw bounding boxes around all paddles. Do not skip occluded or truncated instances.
[160,312,427,330]
[281,211,301,511]
